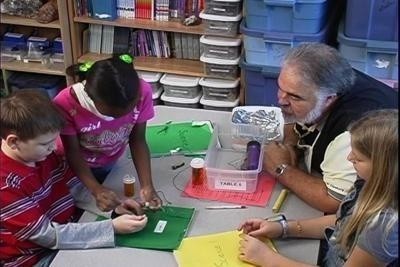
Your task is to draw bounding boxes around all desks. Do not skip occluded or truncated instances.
[49,105,324,267]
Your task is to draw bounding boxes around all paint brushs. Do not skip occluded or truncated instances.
[206,204,248,210]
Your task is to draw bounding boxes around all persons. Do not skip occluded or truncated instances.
[49,52,163,212]
[258,39,399,214]
[0,90,149,267]
[238,109,400,267]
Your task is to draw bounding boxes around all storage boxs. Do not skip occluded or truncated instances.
[200,51,241,80]
[344,0,398,41]
[199,77,241,102]
[204,124,267,193]
[200,95,240,112]
[137,70,162,94]
[200,34,242,60]
[336,19,398,80]
[152,87,163,105]
[199,8,244,37]
[243,0,329,33]
[239,17,331,66]
[238,48,281,106]
[204,0,242,16]
[161,91,201,108]
[160,72,201,99]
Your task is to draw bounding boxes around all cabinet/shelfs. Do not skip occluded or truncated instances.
[67,0,244,105]
[0,0,75,96]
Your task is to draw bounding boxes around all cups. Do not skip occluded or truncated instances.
[190,158,204,188]
[123,175,135,197]
[246,140,260,170]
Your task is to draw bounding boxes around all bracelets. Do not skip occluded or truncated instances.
[296,218,304,239]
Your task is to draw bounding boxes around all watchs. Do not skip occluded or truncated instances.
[269,162,290,181]
[279,219,289,240]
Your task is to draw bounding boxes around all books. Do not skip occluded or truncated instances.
[86,26,201,63]
[74,0,204,22]
